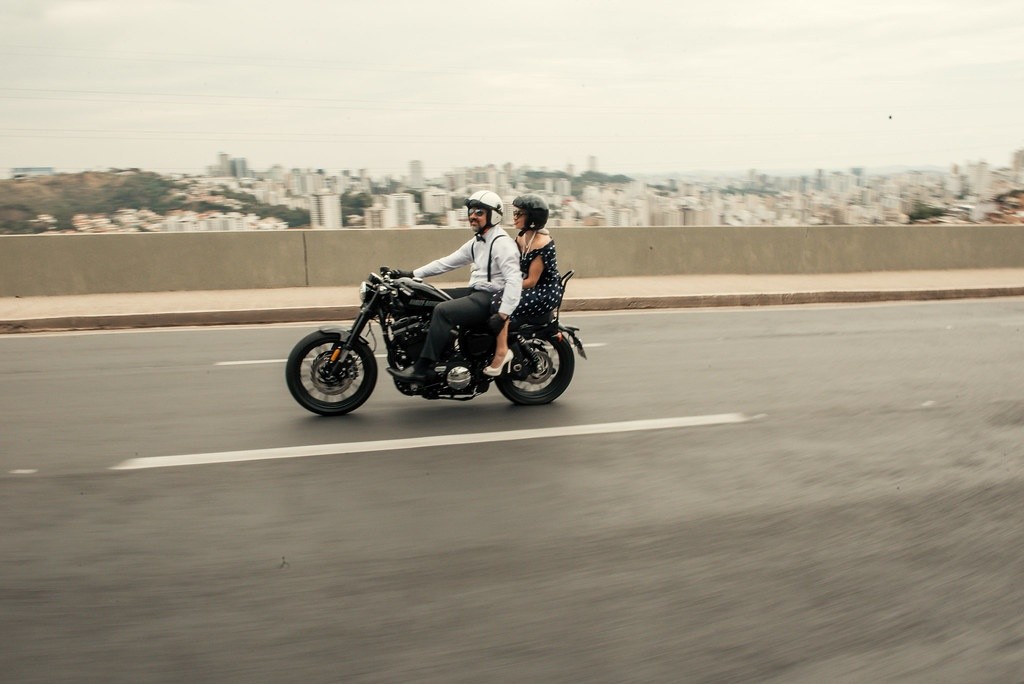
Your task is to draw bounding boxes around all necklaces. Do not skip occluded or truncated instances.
[522,231,536,260]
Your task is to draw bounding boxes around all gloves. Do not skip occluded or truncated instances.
[487,314,505,337]
[396,270,413,279]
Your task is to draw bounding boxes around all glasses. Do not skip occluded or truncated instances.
[513,211,527,217]
[468,209,483,216]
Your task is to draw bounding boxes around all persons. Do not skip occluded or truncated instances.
[483,193,562,376]
[385,189,523,384]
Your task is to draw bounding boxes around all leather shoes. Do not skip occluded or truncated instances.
[387,366,425,381]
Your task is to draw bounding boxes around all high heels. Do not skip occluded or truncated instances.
[483,349,513,376]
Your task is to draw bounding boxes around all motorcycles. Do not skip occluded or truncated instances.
[285,266,587,417]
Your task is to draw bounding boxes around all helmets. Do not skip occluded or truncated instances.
[464,190,504,226]
[513,194,548,230]
